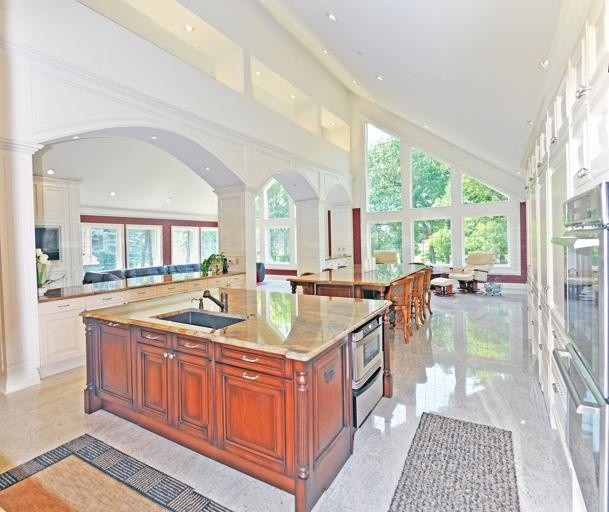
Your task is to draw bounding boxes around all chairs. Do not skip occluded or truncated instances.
[373,250,400,264]
[449,251,494,294]
[384,267,433,343]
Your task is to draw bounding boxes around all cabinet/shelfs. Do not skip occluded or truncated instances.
[130,324,213,459]
[214,337,354,511]
[83,317,133,424]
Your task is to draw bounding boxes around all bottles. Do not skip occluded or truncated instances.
[222,258,228,272]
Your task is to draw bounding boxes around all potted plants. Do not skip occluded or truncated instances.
[201,253,226,277]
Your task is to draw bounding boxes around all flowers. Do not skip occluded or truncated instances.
[36,248,49,284]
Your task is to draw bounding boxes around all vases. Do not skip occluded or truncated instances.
[37,272,45,288]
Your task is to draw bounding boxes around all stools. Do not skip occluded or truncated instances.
[431,277,460,296]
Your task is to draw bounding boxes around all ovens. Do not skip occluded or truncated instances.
[350,315,385,429]
[548,185,609,512]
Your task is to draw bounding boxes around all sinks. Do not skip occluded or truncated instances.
[150,308,249,330]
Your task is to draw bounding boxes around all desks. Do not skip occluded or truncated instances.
[286,264,427,300]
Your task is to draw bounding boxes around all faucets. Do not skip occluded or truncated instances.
[203,289,228,313]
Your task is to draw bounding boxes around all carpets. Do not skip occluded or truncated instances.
[388,410,519,512]
[0,433,234,512]
